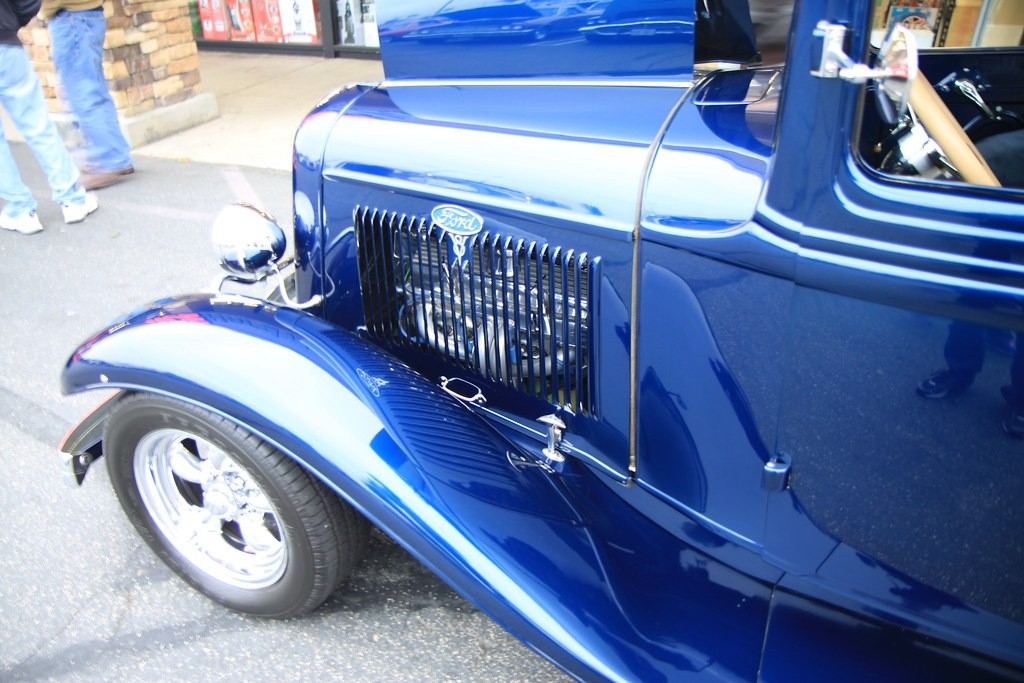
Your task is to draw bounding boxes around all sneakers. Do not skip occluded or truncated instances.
[2,208,41,234]
[60,192,98,224]
[79,165,132,190]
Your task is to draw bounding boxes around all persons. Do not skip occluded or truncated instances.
[0,0,101,235]
[915,190,1024,436]
[36,0,135,192]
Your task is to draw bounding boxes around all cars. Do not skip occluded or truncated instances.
[61,0,1024,683]
